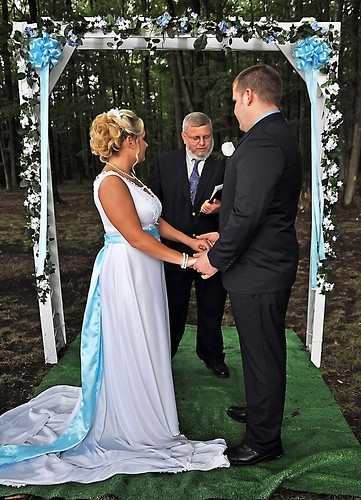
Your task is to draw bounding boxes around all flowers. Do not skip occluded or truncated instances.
[5,10,346,305]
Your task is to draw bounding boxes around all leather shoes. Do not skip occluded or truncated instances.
[227,406,247,424]
[222,442,283,466]
[206,362,230,378]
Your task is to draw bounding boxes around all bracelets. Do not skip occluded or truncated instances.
[181,253,188,269]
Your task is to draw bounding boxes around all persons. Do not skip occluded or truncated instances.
[80,109,213,456]
[193,64,303,466]
[151,112,230,378]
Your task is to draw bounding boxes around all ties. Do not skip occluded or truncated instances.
[189,158,201,205]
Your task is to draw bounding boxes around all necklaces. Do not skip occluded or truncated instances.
[105,162,136,180]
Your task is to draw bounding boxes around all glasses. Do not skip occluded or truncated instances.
[184,132,213,142]
